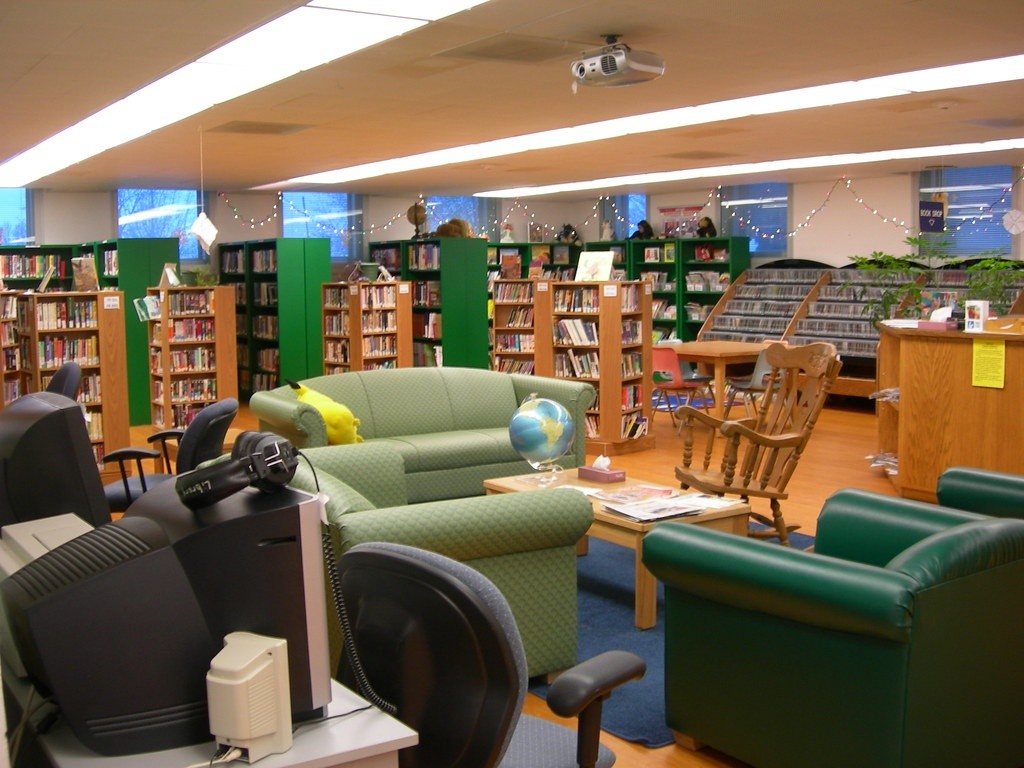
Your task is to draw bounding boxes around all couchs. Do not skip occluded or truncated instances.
[643,488,1024,768]
[248,367,597,510]
[292,439,592,686]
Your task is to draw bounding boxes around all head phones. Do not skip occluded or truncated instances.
[176,429,299,510]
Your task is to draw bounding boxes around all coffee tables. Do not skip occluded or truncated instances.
[479,466,751,627]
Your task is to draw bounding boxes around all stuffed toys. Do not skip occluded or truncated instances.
[625,220,654,241]
[599,218,616,241]
[283,378,365,445]
[693,217,717,237]
[558,223,582,246]
[437,218,470,237]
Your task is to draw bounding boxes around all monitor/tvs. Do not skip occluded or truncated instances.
[0,469,333,757]
[1,392,114,531]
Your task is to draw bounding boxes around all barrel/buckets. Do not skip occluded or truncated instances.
[360,263,380,281]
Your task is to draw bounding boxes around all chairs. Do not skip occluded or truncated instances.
[649,347,709,428]
[723,351,788,421]
[44,361,82,401]
[335,543,646,767]
[673,342,844,548]
[101,396,240,511]
[658,361,716,410]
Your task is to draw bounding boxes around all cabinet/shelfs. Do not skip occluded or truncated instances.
[2,238,1024,459]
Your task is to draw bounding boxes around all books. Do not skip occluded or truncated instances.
[133,295,160,322]
[151,290,217,430]
[362,287,395,308]
[237,344,248,368]
[257,348,279,372]
[0,250,118,471]
[372,244,442,367]
[221,250,276,272]
[237,314,277,340]
[252,373,278,395]
[239,369,249,394]
[325,311,396,375]
[488,245,1023,468]
[325,288,349,308]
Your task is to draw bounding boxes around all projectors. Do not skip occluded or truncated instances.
[570,47,666,86]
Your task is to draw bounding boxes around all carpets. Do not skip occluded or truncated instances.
[651,391,744,413]
[525,520,819,748]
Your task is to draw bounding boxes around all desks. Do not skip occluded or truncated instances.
[658,339,779,434]
[3,626,422,768]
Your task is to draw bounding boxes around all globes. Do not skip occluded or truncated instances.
[509,392,577,486]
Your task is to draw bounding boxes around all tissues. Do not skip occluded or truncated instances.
[577,454,626,484]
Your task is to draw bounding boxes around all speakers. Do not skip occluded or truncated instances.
[207,631,292,764]
